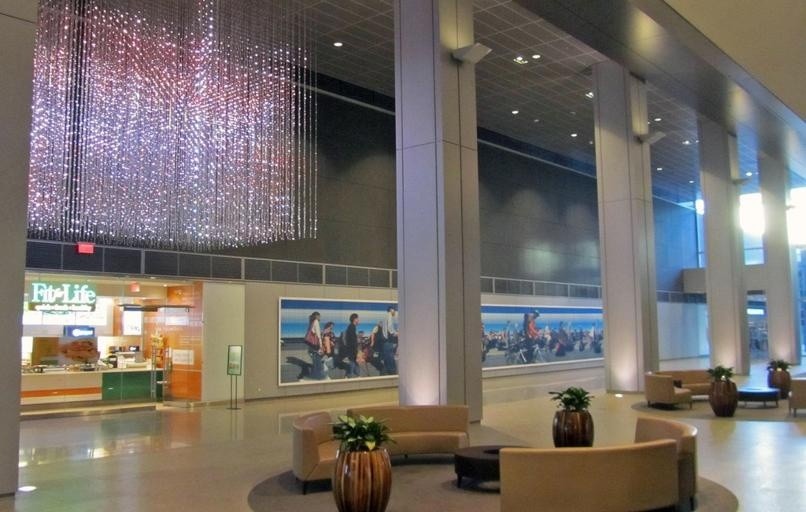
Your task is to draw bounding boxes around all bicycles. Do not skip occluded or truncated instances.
[505,345,553,365]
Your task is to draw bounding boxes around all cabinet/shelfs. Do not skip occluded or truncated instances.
[116,352,143,367]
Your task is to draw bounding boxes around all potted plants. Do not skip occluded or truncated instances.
[766,360,791,400]
[705,365,740,416]
[329,412,392,512]
[548,387,597,447]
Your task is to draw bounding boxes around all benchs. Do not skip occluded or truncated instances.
[291,402,471,498]
[787,376,806,417]
[644,370,721,410]
[498,413,700,512]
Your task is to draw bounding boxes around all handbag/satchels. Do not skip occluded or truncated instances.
[304,330,320,349]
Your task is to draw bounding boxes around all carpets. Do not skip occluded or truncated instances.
[631,398,806,421]
[248,458,739,511]
